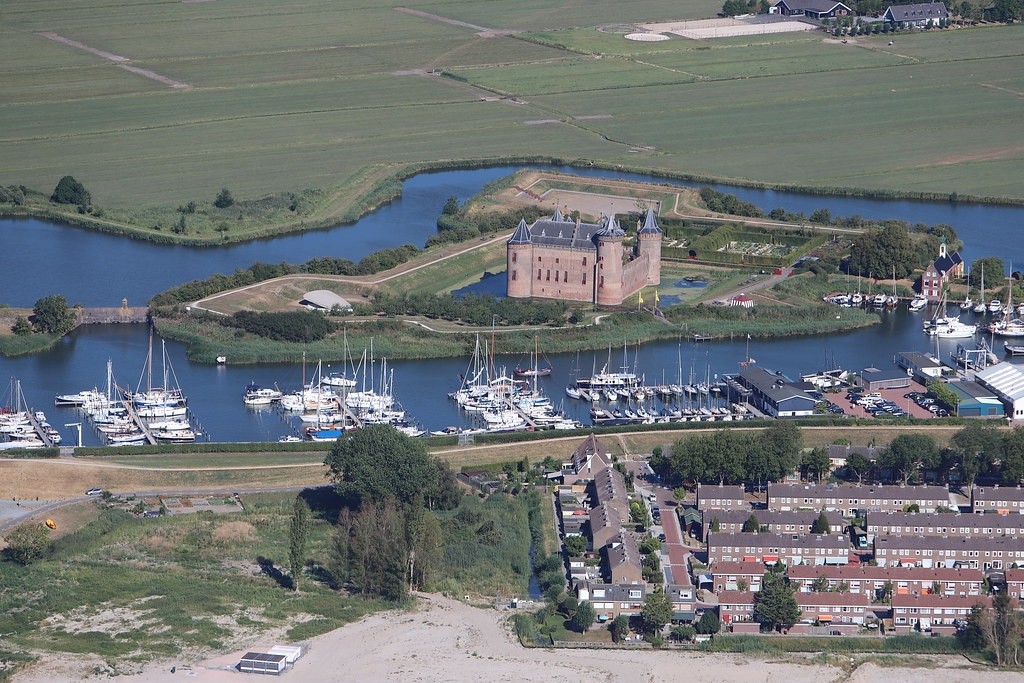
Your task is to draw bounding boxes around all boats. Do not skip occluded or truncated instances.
[1002,305,1014,314]
[987,256,1024,335]
[908,293,928,312]
[989,299,1001,313]
[1003,341,1024,356]
[1018,301,1024,314]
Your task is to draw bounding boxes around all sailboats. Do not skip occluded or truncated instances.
[960,267,972,311]
[429,312,584,435]
[86,487,102,495]
[0,375,62,450]
[242,325,428,440]
[799,346,856,386]
[923,290,977,339]
[825,264,899,308]
[54,324,195,446]
[973,261,987,314]
[566,332,755,425]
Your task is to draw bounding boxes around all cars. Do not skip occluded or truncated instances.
[844,387,913,417]
[814,393,844,414]
[904,391,952,417]
